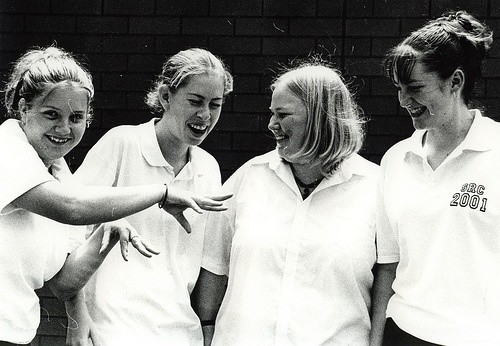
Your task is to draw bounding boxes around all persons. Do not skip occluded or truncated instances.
[0,10,500,346]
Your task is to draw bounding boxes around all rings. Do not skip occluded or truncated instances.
[129,232,138,241]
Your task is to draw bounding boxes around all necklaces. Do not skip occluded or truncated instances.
[294,176,324,200]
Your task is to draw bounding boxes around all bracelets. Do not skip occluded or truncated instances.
[158,183,168,209]
[201,318,215,327]
